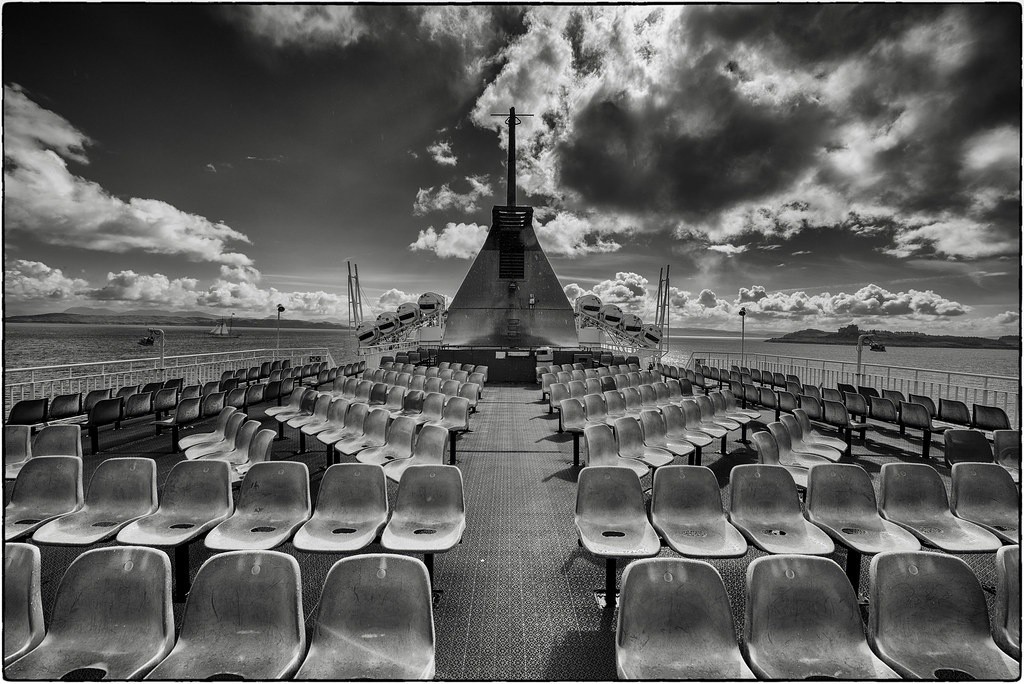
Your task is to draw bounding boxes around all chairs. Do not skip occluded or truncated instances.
[380,464,467,605]
[574,466,662,609]
[804,463,922,606]
[292,554,437,680]
[729,465,836,558]
[534,351,1019,547]
[292,463,389,556]
[203,461,312,551]
[115,460,234,604]
[742,545,1020,680]
[4,542,46,667]
[615,557,756,679]
[31,457,158,548]
[4,347,488,492]
[143,550,306,679]
[948,462,1020,596]
[648,465,748,560]
[5,455,85,542]
[4,546,175,680]
[877,462,1002,555]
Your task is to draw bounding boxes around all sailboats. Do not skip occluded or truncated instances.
[204,314,239,337]
